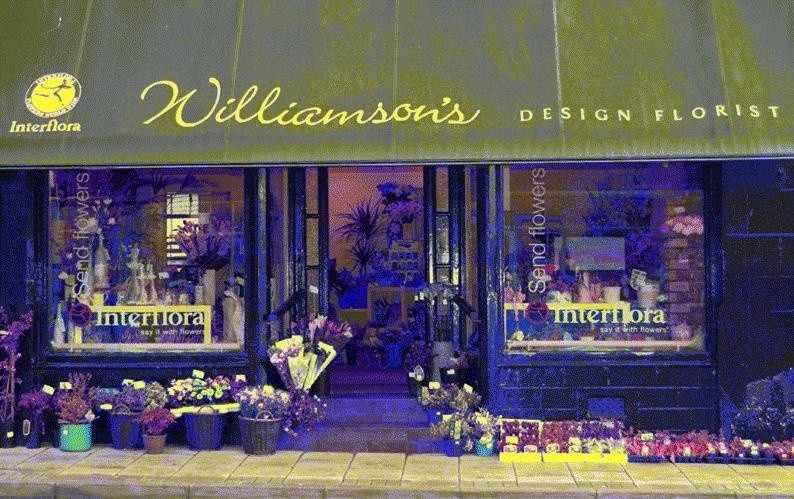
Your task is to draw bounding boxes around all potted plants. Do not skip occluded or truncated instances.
[506,186,707,349]
[168,379,240,451]
[1,309,39,449]
[233,387,293,456]
[101,379,168,451]
[17,387,53,449]
[330,186,481,399]
[50,372,100,452]
[421,390,793,459]
[136,408,176,454]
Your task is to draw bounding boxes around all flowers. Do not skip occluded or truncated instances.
[47,188,243,348]
[269,315,352,428]
